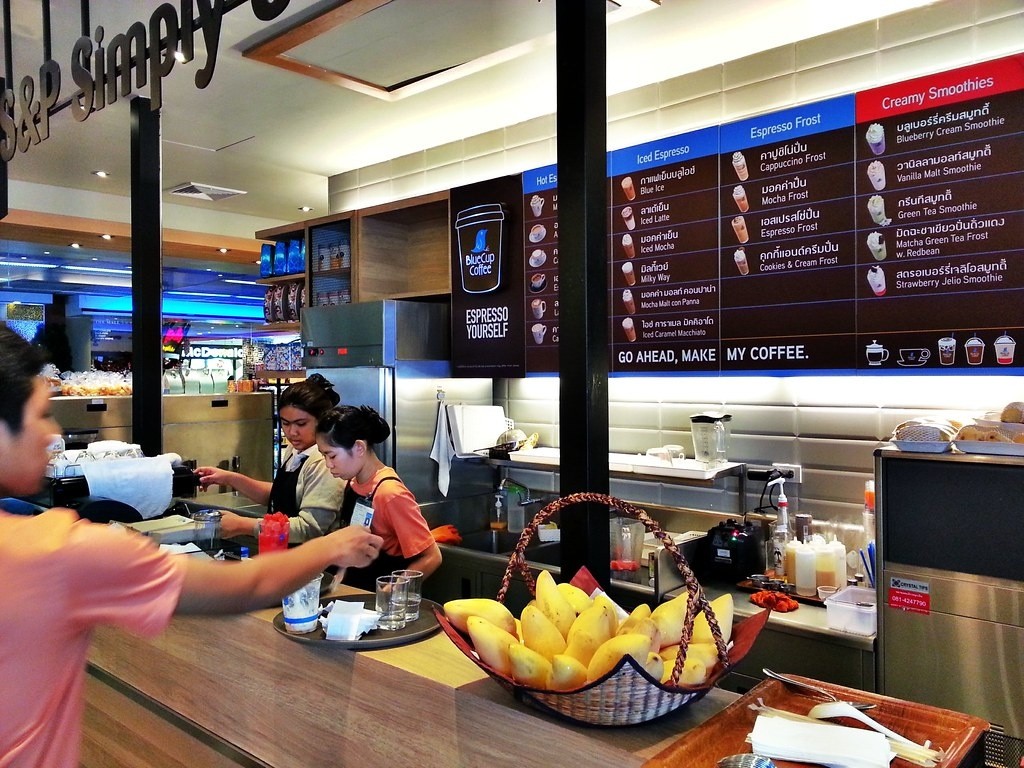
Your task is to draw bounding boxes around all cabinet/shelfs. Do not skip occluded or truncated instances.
[258,378,294,482]
[255,189,451,377]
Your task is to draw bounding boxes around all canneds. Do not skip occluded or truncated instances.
[317,239,351,271]
[317,290,351,307]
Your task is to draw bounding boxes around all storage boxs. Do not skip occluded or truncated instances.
[824,585,876,636]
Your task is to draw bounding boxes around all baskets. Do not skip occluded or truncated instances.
[428,492,771,728]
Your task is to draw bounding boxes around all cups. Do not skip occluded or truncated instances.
[530,127,887,344]
[259,532,289,553]
[645,445,686,468]
[316,238,351,305]
[375,570,423,631]
[193,508,222,551]
[281,572,325,635]
[817,586,839,599]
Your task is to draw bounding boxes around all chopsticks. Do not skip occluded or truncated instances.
[761,707,940,763]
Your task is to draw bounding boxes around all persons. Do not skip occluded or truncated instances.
[192,371,348,546]
[364,518,370,525]
[0,320,386,768]
[314,404,442,592]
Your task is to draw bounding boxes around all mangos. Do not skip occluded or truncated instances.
[895,402,1024,442]
[444,569,734,690]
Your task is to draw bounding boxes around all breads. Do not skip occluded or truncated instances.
[46,376,131,396]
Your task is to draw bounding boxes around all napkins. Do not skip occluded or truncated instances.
[747,717,896,768]
[159,542,202,555]
[319,599,381,641]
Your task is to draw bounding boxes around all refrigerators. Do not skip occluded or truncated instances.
[300,300,493,603]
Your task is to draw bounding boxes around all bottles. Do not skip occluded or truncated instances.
[507,485,525,533]
[785,533,847,597]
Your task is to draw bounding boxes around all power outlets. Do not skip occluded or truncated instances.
[773,463,802,483]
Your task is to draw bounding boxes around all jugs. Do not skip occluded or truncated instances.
[689,412,733,468]
[611,518,646,571]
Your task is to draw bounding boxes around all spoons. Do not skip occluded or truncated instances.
[807,700,917,743]
[763,668,878,710]
